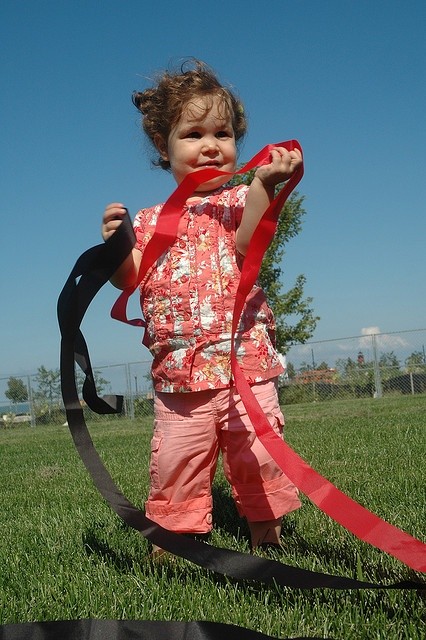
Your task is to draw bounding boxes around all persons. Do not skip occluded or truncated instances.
[100,55,303,597]
